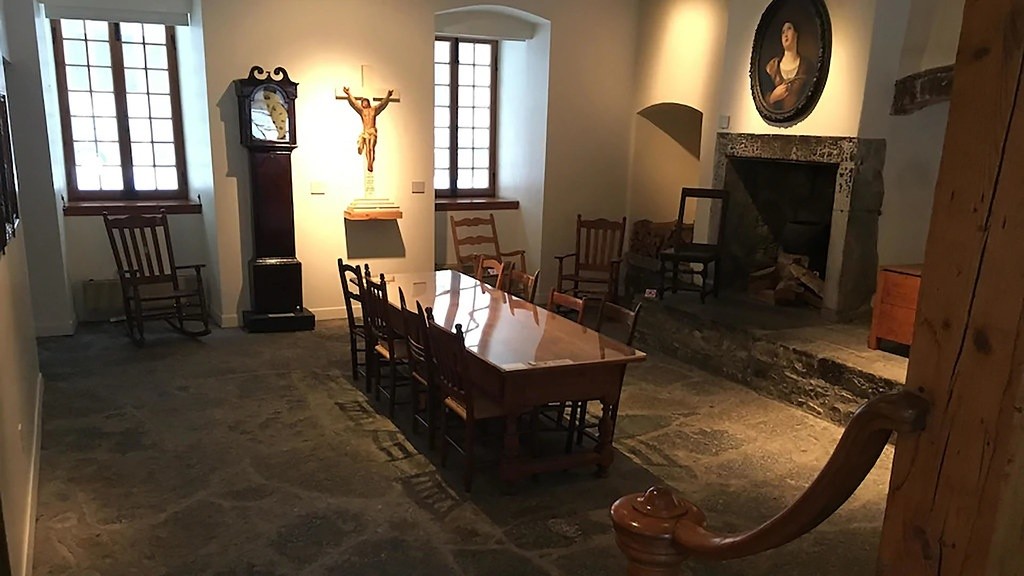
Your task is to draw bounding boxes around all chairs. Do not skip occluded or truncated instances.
[659,188,728,304]
[554,214,627,310]
[450,213,528,299]
[103,208,212,347]
[337,256,642,492]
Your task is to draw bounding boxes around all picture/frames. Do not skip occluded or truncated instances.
[749,0,833,128]
[0,93,21,252]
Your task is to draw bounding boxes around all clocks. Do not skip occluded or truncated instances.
[233,65,315,333]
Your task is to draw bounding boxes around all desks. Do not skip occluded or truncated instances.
[350,269,647,487]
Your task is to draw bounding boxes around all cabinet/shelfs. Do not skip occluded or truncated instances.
[868,264,922,350]
